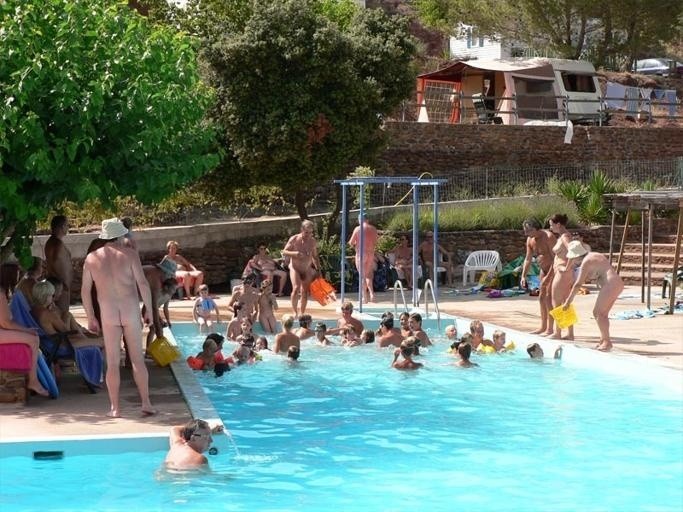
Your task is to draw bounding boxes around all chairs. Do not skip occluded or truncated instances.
[40,326,97,396]
[319,249,503,287]
[472,93,504,125]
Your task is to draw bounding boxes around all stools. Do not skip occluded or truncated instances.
[1,342,32,407]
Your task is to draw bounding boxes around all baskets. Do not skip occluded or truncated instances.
[549,303,578,330]
[148,337,181,367]
[311,278,334,306]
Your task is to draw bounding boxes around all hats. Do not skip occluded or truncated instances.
[156,255,176,278]
[261,279,273,289]
[99,217,128,240]
[566,240,587,259]
[381,318,393,328]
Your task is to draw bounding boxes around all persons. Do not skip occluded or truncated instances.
[157,421,236,472]
[1,211,622,419]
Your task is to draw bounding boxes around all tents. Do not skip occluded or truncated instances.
[416,56,563,126]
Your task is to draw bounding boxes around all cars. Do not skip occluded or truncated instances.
[619,58,683,80]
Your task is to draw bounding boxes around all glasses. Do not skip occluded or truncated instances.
[192,433,212,443]
